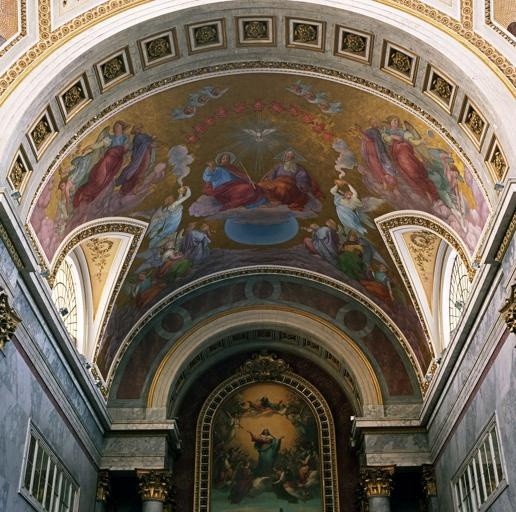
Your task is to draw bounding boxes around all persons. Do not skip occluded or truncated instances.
[213,427,320,502]
[201,152,260,212]
[56,120,213,266]
[303,114,463,307]
[258,148,313,214]
